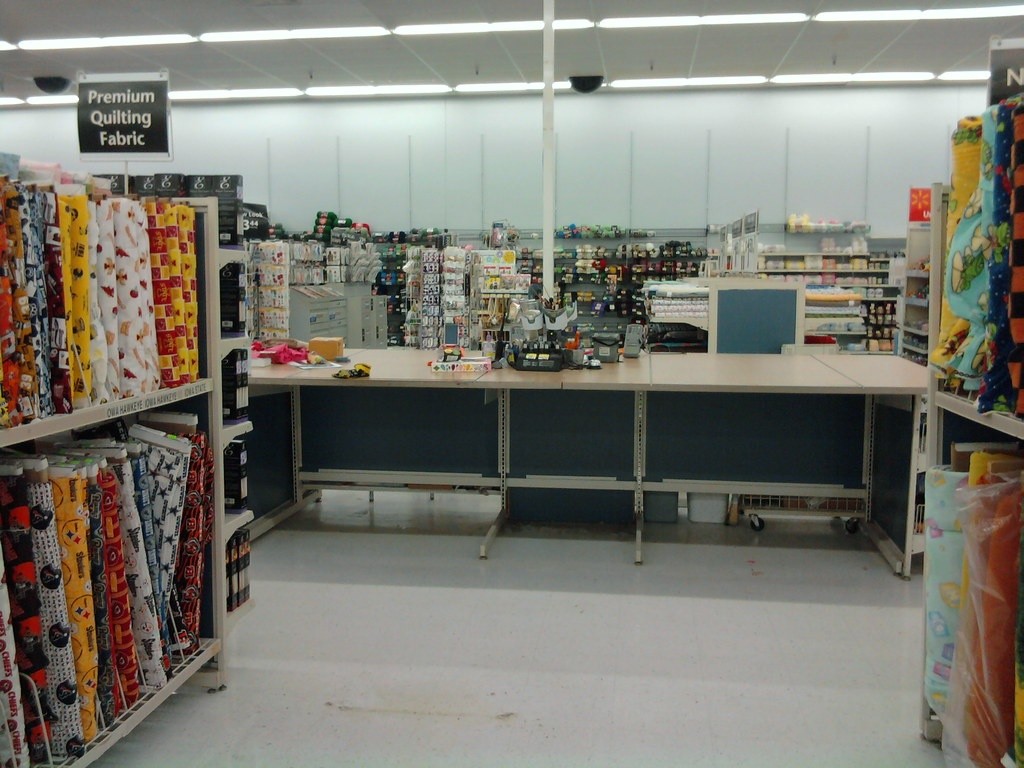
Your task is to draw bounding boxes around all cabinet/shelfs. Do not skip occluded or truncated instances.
[917,182,1024,768]
[897,183,931,366]
[864,258,898,355]
[0,195,254,768]
[720,209,871,336]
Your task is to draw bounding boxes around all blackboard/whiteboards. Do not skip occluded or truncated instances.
[708,275,807,355]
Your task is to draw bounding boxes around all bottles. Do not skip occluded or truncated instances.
[481,331,496,362]
[496,331,504,361]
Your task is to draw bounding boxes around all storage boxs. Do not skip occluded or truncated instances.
[224,440,249,515]
[431,356,492,373]
[239,349,248,422]
[220,262,246,337]
[225,529,250,612]
[309,337,343,362]
[93,175,244,246]
[222,349,242,426]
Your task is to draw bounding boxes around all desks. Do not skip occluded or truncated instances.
[248,349,928,582]
